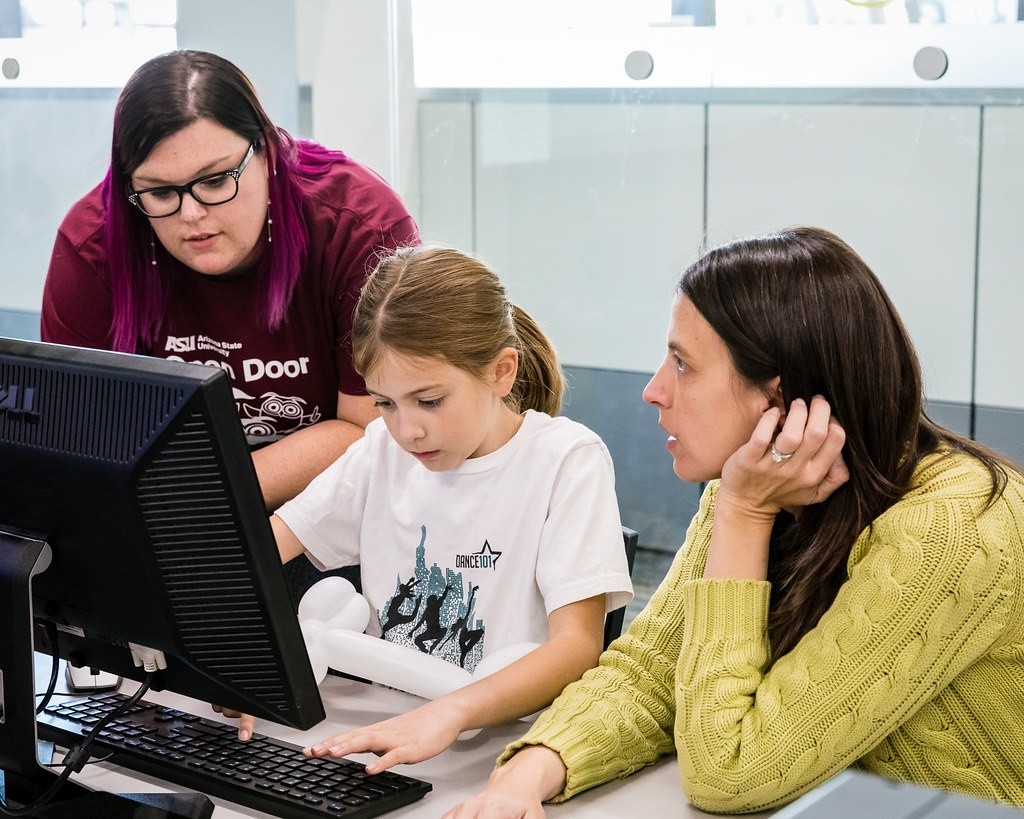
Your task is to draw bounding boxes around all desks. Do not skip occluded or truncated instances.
[49,637,776,819]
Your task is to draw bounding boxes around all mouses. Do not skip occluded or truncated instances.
[65,660,123,694]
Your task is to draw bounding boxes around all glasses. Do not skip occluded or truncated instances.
[125,137,261,219]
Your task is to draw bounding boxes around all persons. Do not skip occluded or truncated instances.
[442,226,1023,819]
[211,244,633,775]
[38,47,423,517]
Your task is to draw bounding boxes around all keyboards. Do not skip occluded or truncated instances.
[35,691,433,819]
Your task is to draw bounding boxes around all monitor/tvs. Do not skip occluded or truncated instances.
[0,335,328,819]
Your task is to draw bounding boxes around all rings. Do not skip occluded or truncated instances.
[770,441,797,466]
